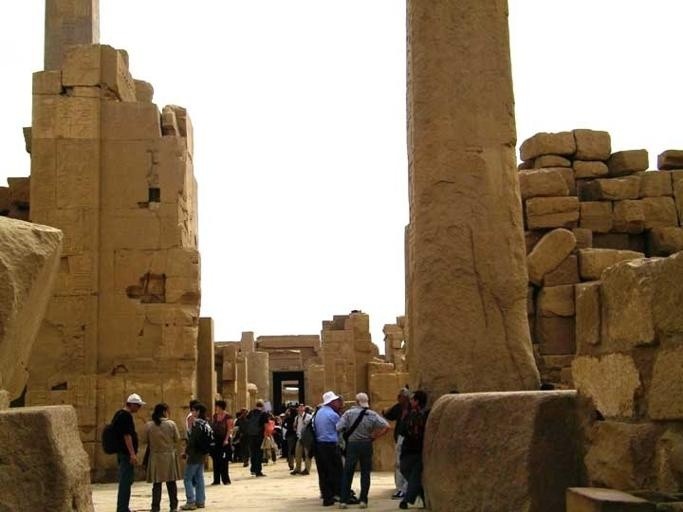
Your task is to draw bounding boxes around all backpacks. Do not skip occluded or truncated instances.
[198,420,216,455]
[102,423,119,455]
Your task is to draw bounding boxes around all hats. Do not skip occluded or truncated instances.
[356,392,370,408]
[317,391,340,407]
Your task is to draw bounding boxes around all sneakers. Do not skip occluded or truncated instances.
[359,501,366,508]
[335,502,346,509]
[197,503,205,508]
[180,503,196,510]
[391,490,406,499]
[291,470,301,475]
[400,501,408,508]
[300,469,310,474]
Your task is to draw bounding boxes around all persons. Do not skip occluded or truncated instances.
[233,391,359,506]
[398,390,432,509]
[144,402,179,511]
[381,387,411,496]
[185,400,200,486]
[210,400,233,485]
[449,390,460,394]
[179,403,211,510]
[335,392,391,508]
[110,393,146,512]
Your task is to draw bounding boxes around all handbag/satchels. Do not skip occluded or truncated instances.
[336,432,346,455]
[300,419,316,458]
